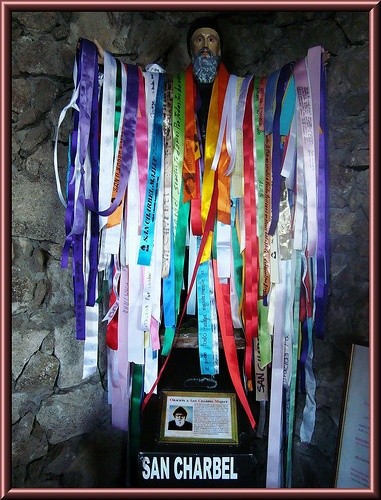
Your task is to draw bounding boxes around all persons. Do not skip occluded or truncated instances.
[167,406,193,432]
[78,15,333,321]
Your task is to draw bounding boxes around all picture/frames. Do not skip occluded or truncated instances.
[0,0,381,500]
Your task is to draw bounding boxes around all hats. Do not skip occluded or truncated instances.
[173,406,187,416]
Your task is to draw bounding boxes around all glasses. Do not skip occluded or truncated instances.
[175,415,185,419]
